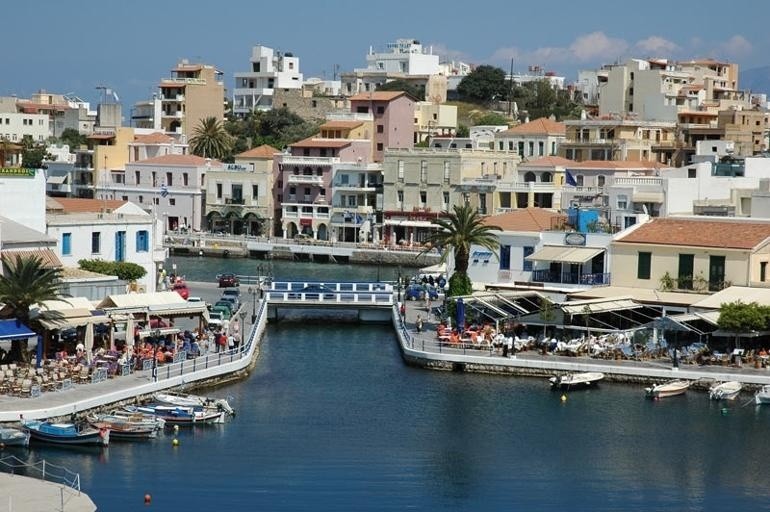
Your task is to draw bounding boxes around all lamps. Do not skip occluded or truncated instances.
[2,339,210,399]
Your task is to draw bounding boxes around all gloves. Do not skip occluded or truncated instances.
[755,384,770,405]
[0,421,31,450]
[19,412,111,446]
[645,379,692,399]
[549,371,604,388]
[85,411,159,438]
[154,390,235,416]
[707,380,743,400]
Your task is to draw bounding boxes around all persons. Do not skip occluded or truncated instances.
[54,318,240,376]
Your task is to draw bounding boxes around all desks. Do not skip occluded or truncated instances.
[150,328,161,381]
[507,310,521,359]
[239,309,249,353]
[248,284,259,325]
[255,261,265,302]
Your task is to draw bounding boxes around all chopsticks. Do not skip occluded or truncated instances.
[116,403,224,425]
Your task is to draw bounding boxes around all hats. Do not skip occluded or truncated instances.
[152,318,169,329]
[289,285,334,299]
[403,284,439,301]
[207,287,241,331]
[219,273,241,287]
[187,297,203,302]
[294,233,313,242]
[60,324,106,340]
[173,282,189,299]
